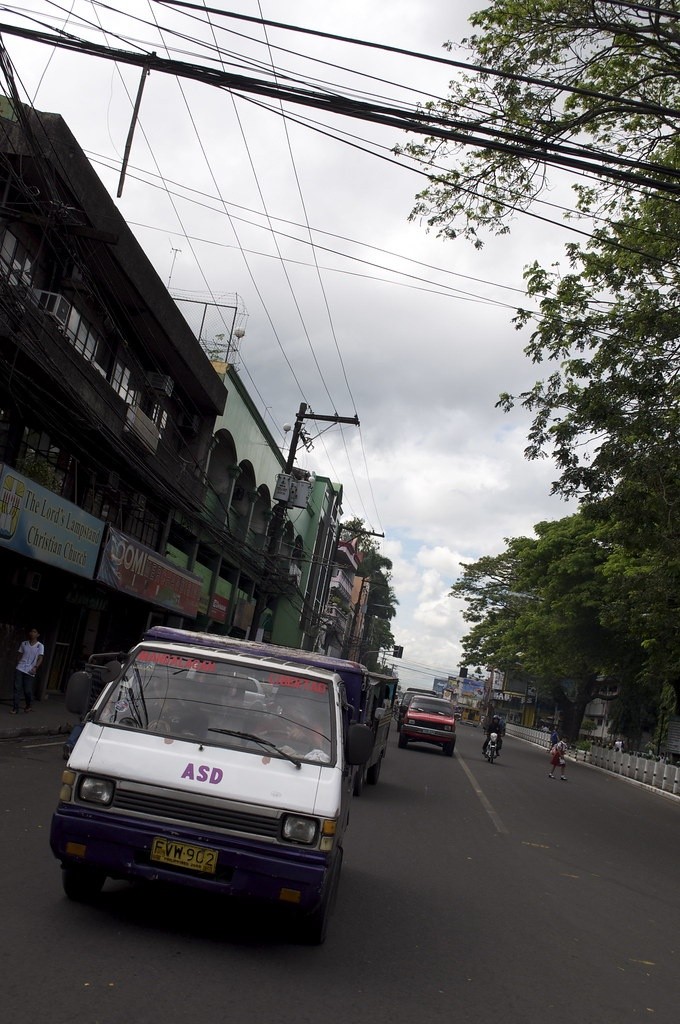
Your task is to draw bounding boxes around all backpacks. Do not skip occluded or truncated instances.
[550,744,557,755]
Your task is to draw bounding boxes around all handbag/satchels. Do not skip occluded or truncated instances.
[559,757,566,765]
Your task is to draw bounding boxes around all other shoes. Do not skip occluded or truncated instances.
[561,776,566,780]
[10,709,19,714]
[549,774,555,778]
[25,707,32,712]
[482,751,486,754]
[496,751,500,756]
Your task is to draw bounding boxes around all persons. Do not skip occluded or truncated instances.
[9,626,45,714]
[250,686,327,750]
[63,651,117,760]
[482,715,506,756]
[548,736,570,780]
[549,726,560,749]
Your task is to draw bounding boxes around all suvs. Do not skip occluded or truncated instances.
[398,687,456,757]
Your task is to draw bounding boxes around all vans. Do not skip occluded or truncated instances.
[49,626,400,947]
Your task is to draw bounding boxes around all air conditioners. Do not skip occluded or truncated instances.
[144,371,175,398]
[29,289,71,326]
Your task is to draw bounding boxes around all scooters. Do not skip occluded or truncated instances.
[481,727,500,764]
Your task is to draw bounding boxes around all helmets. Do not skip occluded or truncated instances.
[492,715,500,719]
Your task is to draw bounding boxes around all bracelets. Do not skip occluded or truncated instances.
[35,666,38,668]
